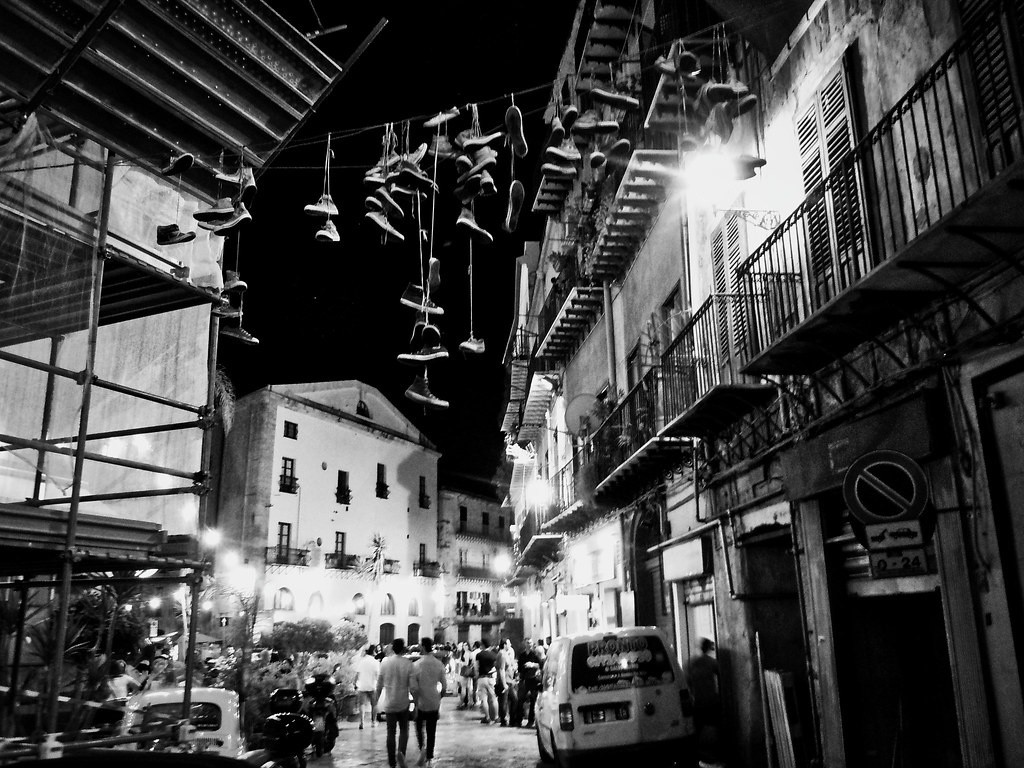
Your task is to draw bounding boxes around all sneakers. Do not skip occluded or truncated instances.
[425,258,441,293]
[211,302,244,317]
[215,166,244,185]
[404,374,449,410]
[193,198,234,220]
[590,87,639,111]
[539,103,632,178]
[655,50,701,81]
[223,270,247,294]
[696,80,758,141]
[502,180,525,233]
[397,340,449,365]
[363,108,502,245]
[220,325,260,345]
[505,106,528,157]
[198,202,252,236]
[234,168,256,210]
[399,282,444,315]
[409,312,442,344]
[459,339,485,353]
[161,154,195,176]
[304,132,338,217]
[155,224,195,245]
[314,215,342,242]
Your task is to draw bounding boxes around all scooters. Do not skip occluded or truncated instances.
[235,711,317,768]
[290,663,343,758]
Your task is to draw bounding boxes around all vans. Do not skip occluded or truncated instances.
[523,626,697,767]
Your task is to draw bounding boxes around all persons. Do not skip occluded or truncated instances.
[374,638,419,768]
[98,636,552,730]
[410,637,448,768]
[681,637,732,764]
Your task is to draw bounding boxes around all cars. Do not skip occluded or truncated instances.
[113,687,245,761]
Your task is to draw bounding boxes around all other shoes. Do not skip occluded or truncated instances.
[388,761,396,768]
[371,721,378,727]
[397,753,408,768]
[481,715,501,723]
[416,748,436,768]
[728,152,767,180]
[359,723,363,729]
